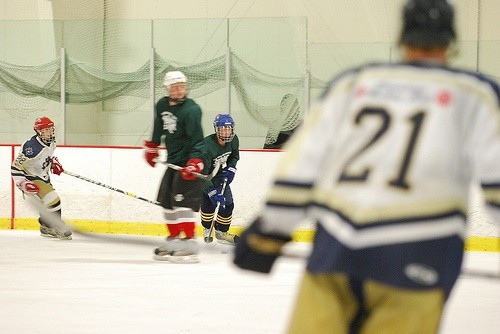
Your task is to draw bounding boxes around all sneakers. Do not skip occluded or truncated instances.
[202,228,214,244]
[213,230,242,247]
[152,236,202,263]
[38,218,73,241]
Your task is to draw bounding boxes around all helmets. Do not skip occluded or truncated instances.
[163,70,187,85]
[32,117,55,133]
[398,0,460,53]
[213,113,235,142]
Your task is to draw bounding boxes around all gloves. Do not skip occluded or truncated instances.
[145,140,160,168]
[207,187,228,210]
[51,159,64,176]
[181,158,205,181]
[213,166,238,186]
[234,218,293,277]
[16,178,40,195]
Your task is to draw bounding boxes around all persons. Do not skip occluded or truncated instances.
[11,117,73,238]
[140,71,208,258]
[231,0,500,334]
[194,111,240,245]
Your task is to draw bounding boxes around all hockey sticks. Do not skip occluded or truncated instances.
[16,182,500,284]
[63,169,175,210]
[207,178,227,248]
[152,157,222,182]
